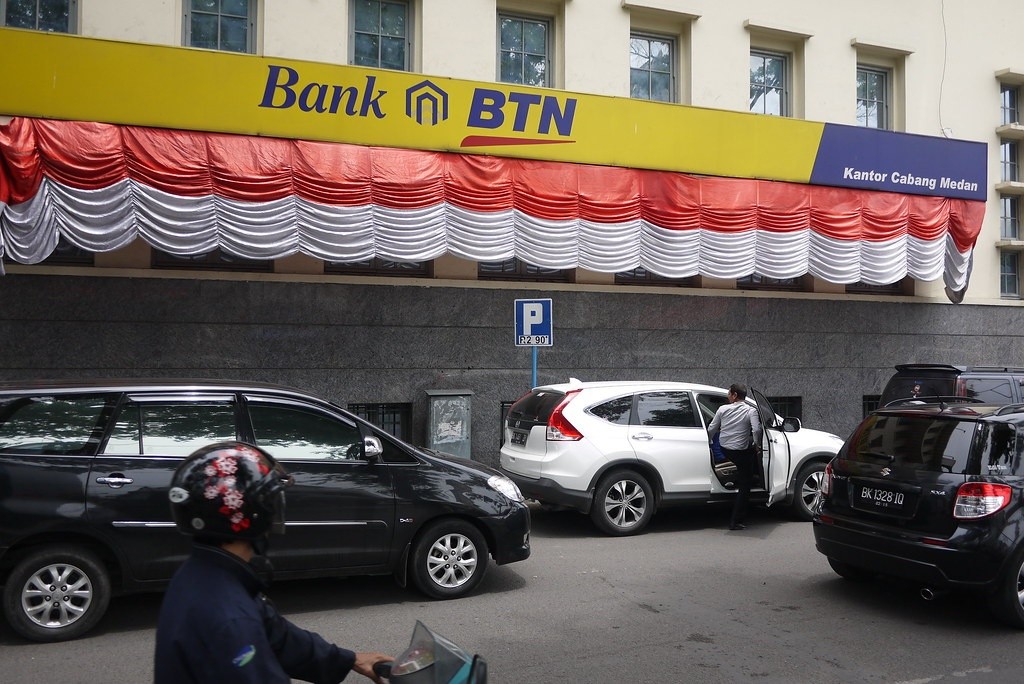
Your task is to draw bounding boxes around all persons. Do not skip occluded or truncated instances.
[706,418,731,465]
[707,383,763,531]
[153,442,397,684]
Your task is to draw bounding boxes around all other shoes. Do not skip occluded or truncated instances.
[730,521,747,530]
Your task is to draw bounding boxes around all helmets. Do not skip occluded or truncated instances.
[169,442,296,550]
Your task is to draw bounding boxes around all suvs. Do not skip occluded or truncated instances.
[0,383,531,645]
[812,395,1024,632]
[499,377,845,538]
[875,362,1024,413]
